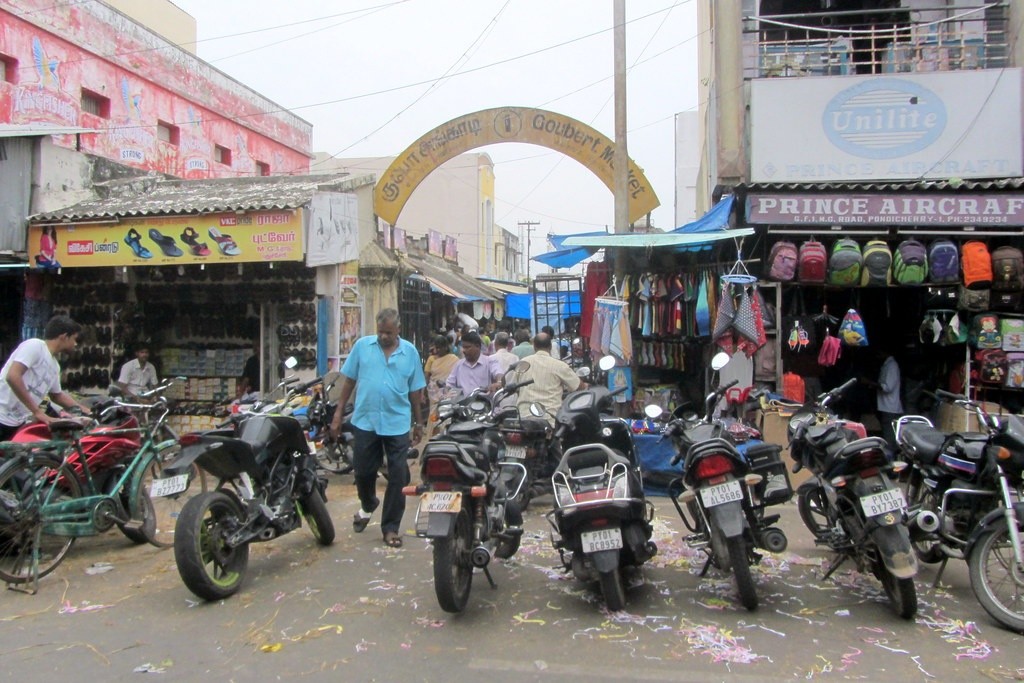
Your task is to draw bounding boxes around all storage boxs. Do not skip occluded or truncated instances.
[935,401,1008,434]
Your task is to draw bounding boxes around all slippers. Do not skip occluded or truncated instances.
[384,533,402,547]
[353,512,370,532]
[149,229,184,257]
[208,226,241,255]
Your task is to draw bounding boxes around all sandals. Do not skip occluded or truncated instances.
[124,229,152,258]
[181,227,210,255]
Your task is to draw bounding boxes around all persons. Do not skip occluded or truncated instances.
[0,315,91,558]
[843,345,905,454]
[423,310,560,441]
[118,346,158,414]
[445,331,506,399]
[511,333,592,476]
[330,308,426,547]
[238,331,285,400]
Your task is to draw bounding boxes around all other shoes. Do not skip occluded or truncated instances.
[41,264,318,391]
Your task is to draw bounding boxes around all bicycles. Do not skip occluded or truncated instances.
[1,375,210,596]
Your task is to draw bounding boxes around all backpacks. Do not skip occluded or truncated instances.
[765,234,1023,389]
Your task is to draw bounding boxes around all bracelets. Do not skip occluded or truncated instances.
[497,381,502,383]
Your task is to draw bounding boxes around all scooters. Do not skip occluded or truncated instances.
[1,386,158,560]
[528,353,657,611]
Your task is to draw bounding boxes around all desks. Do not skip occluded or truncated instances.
[632,434,765,495]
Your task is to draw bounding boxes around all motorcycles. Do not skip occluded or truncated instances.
[783,375,940,619]
[644,352,794,612]
[891,386,1024,633]
[228,353,616,485]
[399,361,535,612]
[150,373,335,602]
